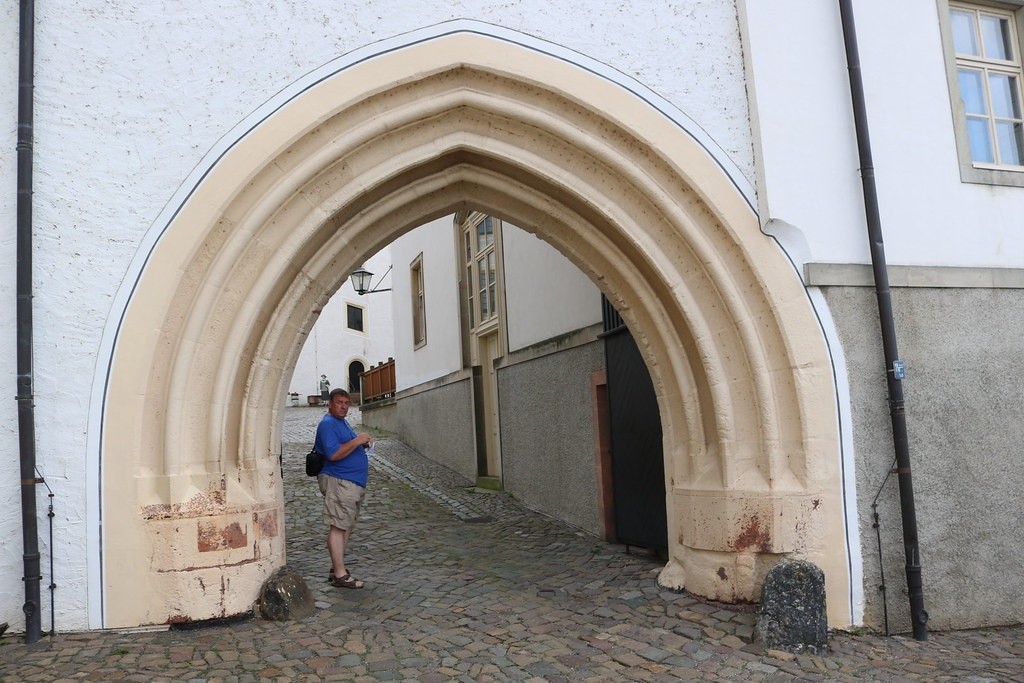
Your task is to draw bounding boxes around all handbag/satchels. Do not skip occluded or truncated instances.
[306,451,324,477]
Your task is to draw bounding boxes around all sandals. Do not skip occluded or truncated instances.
[329,568,350,581]
[331,573,364,589]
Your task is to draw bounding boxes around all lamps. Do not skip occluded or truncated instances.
[350,264,393,296]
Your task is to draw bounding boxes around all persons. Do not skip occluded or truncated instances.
[305,374,374,589]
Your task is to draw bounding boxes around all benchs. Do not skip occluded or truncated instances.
[348,393,360,405]
